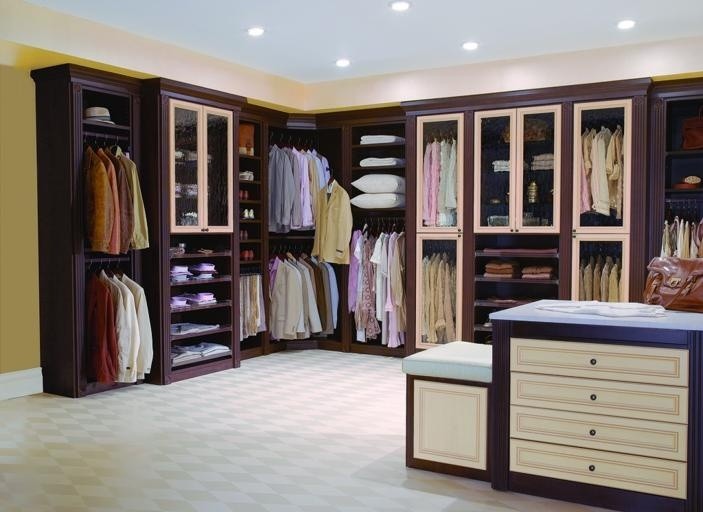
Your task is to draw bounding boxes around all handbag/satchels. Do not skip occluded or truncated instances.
[642,255,703,314]
[678,106,703,151]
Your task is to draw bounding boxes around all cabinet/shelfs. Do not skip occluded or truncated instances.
[321,74,690,365]
[397,339,491,485]
[29,64,319,396]
[485,296,701,510]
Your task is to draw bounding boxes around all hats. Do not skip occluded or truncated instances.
[82,105,116,126]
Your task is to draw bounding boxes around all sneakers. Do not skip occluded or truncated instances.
[240,249,254,262]
[238,189,249,200]
[239,229,248,241]
[238,170,254,182]
[240,207,255,221]
[238,144,254,157]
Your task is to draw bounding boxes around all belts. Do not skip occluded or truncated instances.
[671,174,702,190]
